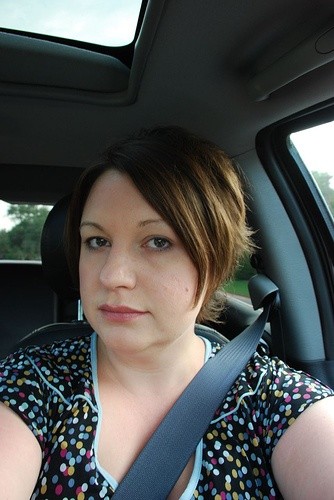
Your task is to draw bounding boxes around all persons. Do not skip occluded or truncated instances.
[0,129,334,500]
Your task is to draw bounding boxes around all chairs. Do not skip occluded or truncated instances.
[11,201,232,350]
